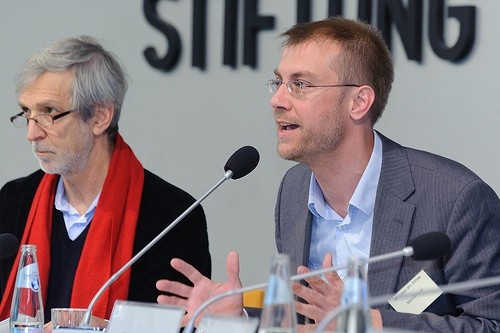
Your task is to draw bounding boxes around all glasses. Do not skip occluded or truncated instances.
[266,76,361,99]
[8,108,76,128]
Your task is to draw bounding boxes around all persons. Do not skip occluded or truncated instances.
[0,35,211,333]
[156,15,500,333]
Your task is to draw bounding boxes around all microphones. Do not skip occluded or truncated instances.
[0,234,19,260]
[184,232,454,333]
[53,146,260,332]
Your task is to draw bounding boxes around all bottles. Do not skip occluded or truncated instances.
[8,244,45,333]
[258,254,299,333]
[339,256,371,333]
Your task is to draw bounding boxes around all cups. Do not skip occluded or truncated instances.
[50,308,91,333]
